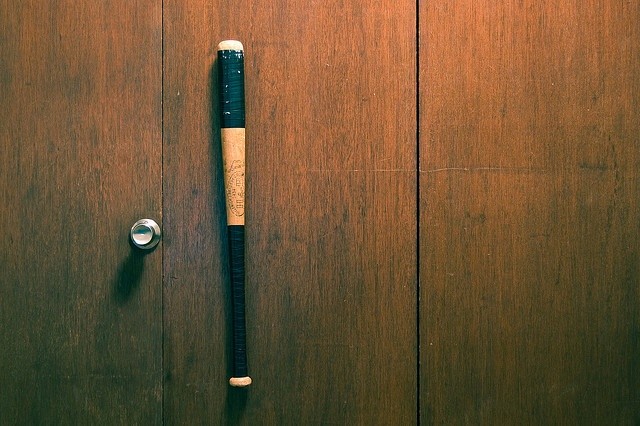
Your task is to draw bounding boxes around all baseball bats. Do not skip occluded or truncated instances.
[218,39,252,388]
